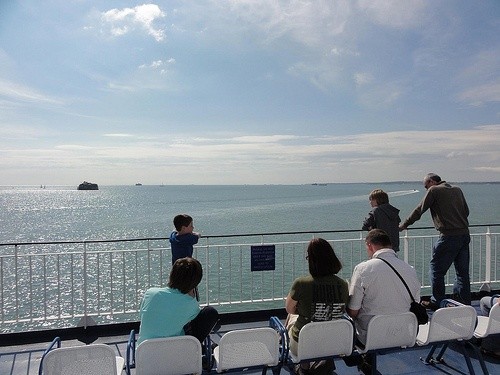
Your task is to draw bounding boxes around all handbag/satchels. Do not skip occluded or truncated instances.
[409,301,430,325]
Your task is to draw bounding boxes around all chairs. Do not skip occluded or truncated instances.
[38,292,500,375]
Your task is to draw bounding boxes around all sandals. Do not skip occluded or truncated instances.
[296,360,337,375]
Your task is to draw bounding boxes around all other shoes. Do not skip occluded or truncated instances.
[421,300,440,311]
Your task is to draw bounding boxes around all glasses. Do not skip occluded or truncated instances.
[306,256,309,260]
[425,186,427,188]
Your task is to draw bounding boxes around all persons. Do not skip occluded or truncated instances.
[480,296,500,317]
[362,190,401,254]
[346,228,421,375]
[137,257,220,347]
[398,173,471,310]
[169,214,200,265]
[283,238,349,375]
